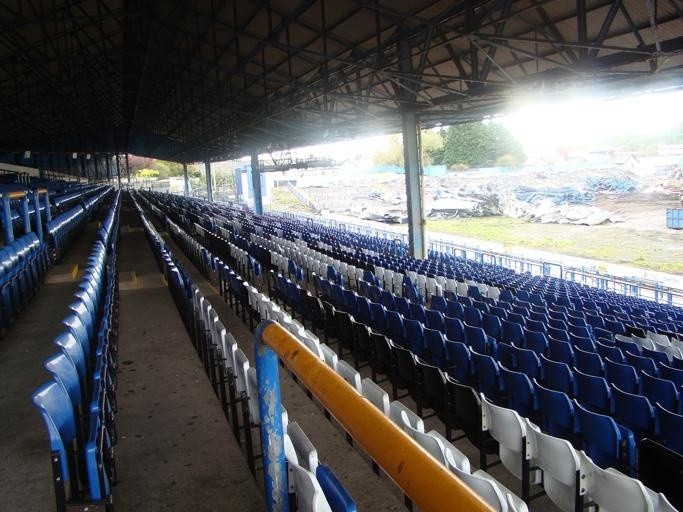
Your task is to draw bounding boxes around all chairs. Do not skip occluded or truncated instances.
[0,163,683,512]
